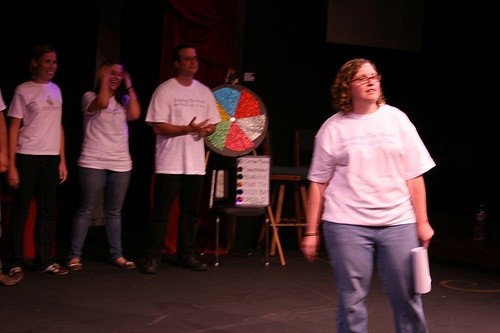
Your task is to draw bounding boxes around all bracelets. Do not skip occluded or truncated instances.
[304,232,320,236]
[127,86,133,90]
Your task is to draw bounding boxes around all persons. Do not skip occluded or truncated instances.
[301,59,437,333]
[0,46,70,275]
[0,88,23,285]
[141,44,220,273]
[63,59,142,270]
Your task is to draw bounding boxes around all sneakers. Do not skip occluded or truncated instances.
[40,263,70,274]
[8,266,23,281]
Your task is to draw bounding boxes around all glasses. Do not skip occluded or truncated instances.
[349,74,382,84]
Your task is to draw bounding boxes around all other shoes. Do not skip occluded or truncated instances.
[144,256,160,274]
[179,255,208,270]
[66,258,83,270]
[0,272,20,286]
[113,256,135,269]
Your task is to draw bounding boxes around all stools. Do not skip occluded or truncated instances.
[209,205,272,268]
[270,165,315,257]
